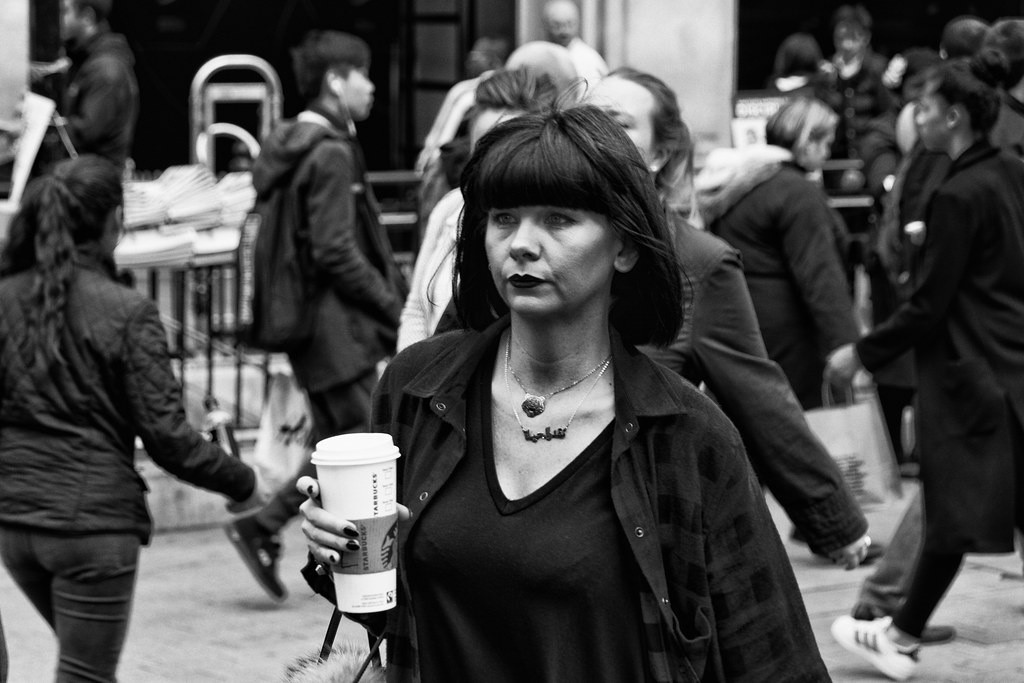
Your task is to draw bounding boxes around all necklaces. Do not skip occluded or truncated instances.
[504,333,611,444]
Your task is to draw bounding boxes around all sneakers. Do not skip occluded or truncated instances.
[221,515,288,602]
[830,615,923,681]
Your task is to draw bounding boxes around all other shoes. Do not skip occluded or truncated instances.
[922,626,957,646]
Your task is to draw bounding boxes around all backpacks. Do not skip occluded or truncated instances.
[232,129,333,352]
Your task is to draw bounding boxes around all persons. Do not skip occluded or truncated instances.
[1,154,273,683]
[223,31,412,601]
[761,33,850,199]
[294,96,835,682]
[0,0,140,189]
[830,5,1024,682]
[597,64,873,572]
[398,64,589,358]
[541,0,609,103]
[693,92,858,413]
[415,40,511,183]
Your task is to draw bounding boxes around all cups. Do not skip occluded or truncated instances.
[310,433,401,612]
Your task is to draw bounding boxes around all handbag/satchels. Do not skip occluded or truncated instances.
[804,363,903,513]
